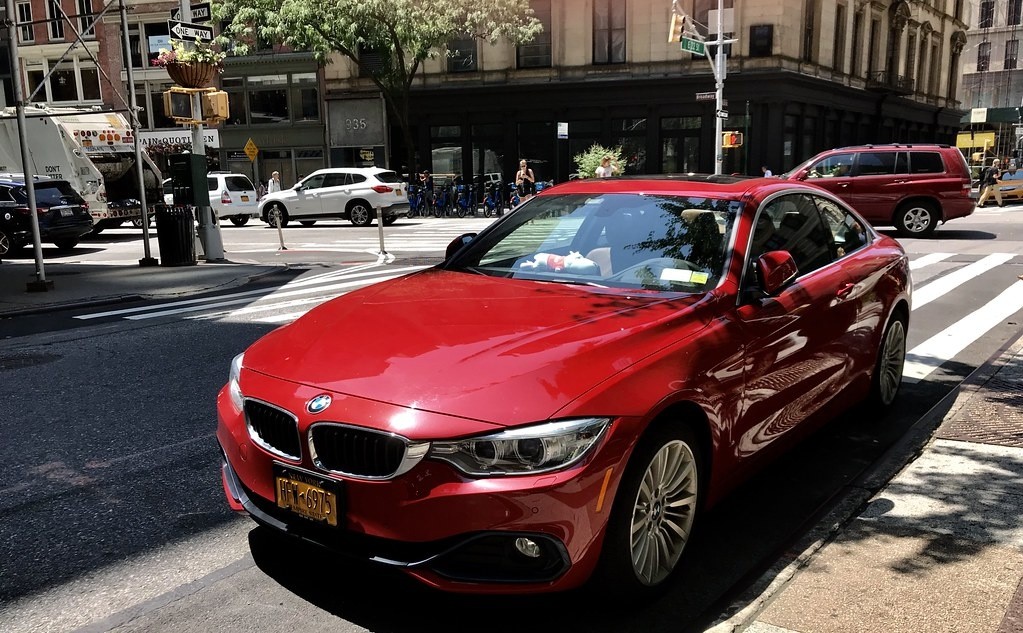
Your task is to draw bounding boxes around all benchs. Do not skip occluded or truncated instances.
[681,208,820,273]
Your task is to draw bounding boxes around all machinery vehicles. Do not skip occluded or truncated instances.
[0,99,166,243]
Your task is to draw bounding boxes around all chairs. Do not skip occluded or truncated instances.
[585,215,632,276]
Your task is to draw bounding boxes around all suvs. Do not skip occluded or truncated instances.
[132,171,257,228]
[258,166,411,227]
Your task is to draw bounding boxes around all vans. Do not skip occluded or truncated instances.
[452,173,503,193]
[779,142,976,236]
[401,173,455,196]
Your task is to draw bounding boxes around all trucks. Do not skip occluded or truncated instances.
[432,147,503,174]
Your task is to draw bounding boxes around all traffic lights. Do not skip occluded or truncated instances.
[722,131,743,148]
[164,90,194,120]
[203,90,229,121]
[972,152,983,162]
[668,13,686,43]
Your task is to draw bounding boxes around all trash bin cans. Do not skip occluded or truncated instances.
[155,204,198,266]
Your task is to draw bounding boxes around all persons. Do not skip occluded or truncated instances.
[762,166,773,177]
[811,158,853,177]
[259,171,303,202]
[594,156,621,178]
[419,170,433,191]
[977,159,1008,207]
[516,159,535,226]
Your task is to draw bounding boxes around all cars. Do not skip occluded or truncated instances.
[0,173,93,259]
[986,168,1023,202]
[216,173,912,608]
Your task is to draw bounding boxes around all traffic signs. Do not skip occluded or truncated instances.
[169,2,212,23]
[168,19,214,45]
[716,110,729,121]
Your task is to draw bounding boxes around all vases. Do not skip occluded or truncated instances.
[166,59,215,88]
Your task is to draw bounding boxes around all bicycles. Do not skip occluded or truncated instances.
[407,180,553,218]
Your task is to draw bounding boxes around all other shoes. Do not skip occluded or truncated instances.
[977,203,1007,207]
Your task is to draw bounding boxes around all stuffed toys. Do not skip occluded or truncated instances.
[520,250,600,273]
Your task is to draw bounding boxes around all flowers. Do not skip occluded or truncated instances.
[150,34,248,76]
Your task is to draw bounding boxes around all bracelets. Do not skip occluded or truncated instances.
[527,176,530,181]
